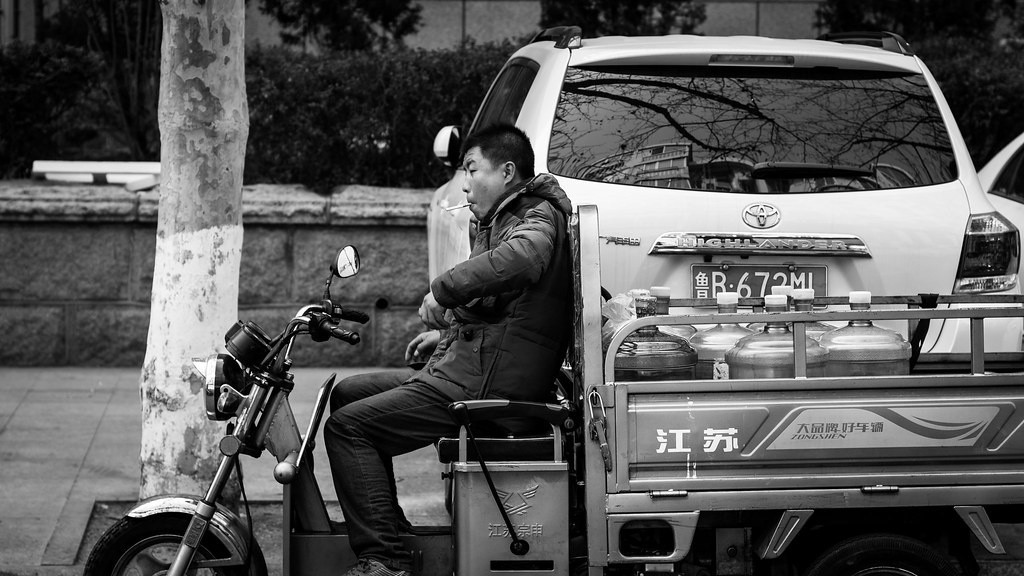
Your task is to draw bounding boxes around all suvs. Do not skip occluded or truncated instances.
[426,25,1024,352]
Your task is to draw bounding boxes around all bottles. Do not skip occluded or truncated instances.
[614,286,913,381]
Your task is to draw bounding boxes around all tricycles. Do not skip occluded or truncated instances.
[82,205,1024,576]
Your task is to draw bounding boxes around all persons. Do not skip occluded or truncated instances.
[323,122,572,576]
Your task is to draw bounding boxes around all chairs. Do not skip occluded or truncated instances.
[435,363,577,462]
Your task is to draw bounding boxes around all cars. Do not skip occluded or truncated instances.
[968,132,1024,282]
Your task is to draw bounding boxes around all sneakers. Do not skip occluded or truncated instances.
[343,557,412,576]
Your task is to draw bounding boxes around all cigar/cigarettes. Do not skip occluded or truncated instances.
[445,203,472,211]
